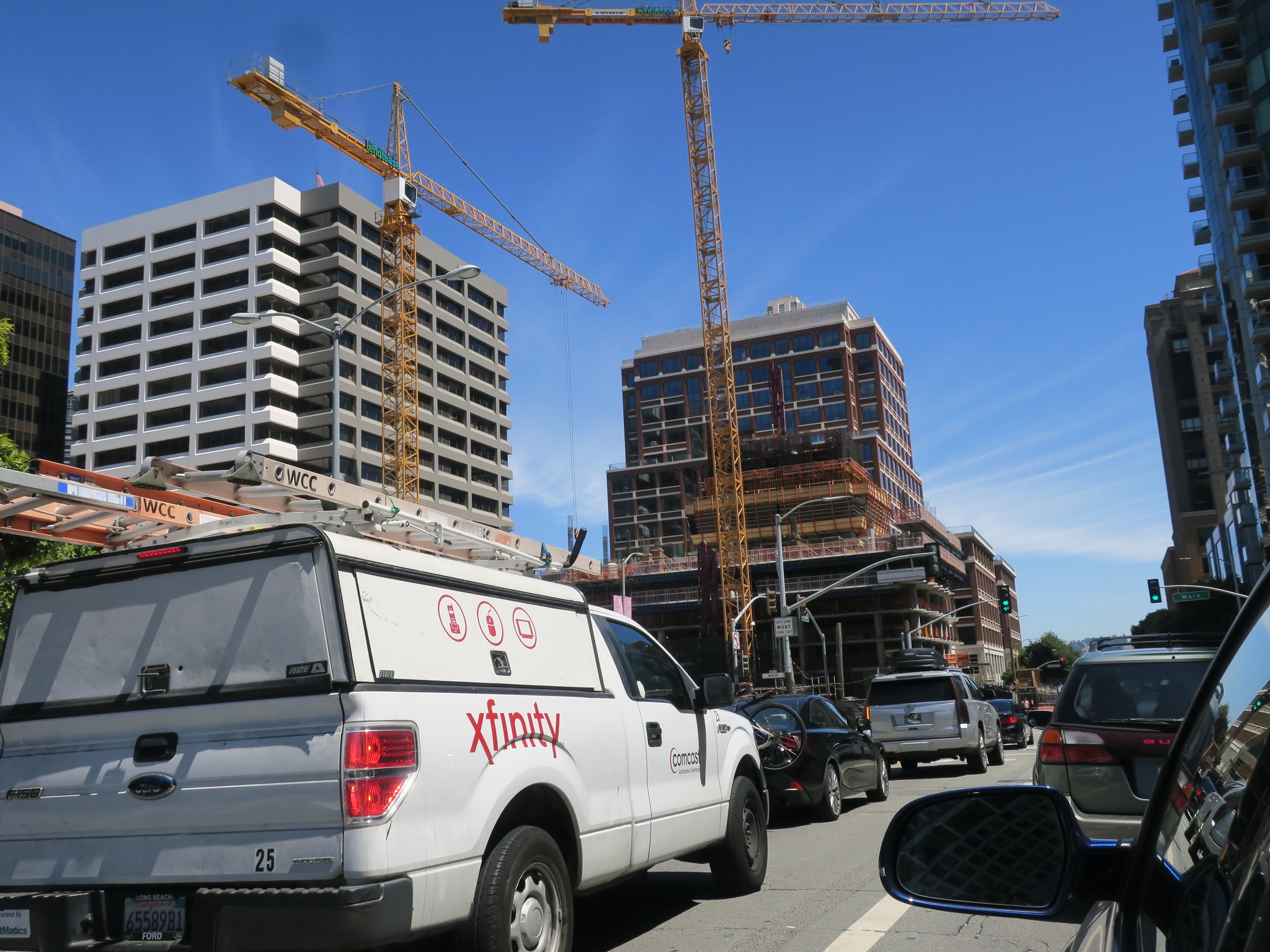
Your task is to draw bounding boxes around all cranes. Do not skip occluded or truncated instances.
[229,57,612,497]
[501,1,1062,667]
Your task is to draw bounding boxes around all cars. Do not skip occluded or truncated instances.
[983,698,1034,748]
[1183,771,1248,858]
[716,693,891,821]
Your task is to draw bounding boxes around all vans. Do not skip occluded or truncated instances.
[0,504,769,952]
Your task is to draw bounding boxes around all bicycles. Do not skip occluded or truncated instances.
[727,689,808,772]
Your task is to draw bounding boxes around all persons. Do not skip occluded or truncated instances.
[1020,698,1030,715]
[1029,698,1035,710]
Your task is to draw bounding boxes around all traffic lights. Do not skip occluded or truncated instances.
[1060,656,1064,669]
[999,584,1013,614]
[1251,689,1270,714]
[766,587,777,615]
[796,594,807,620]
[1147,578,1163,604]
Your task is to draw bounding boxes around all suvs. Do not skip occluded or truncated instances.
[860,647,1005,778]
[1025,632,1228,844]
[873,560,1270,952]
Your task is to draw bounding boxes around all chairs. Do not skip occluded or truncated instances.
[1091,678,1136,718]
[770,714,787,723]
[1153,679,1190,719]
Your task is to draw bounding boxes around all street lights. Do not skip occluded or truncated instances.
[622,552,644,616]
[773,494,851,694]
[1014,637,1035,670]
[228,262,484,482]
[1007,615,1028,689]
